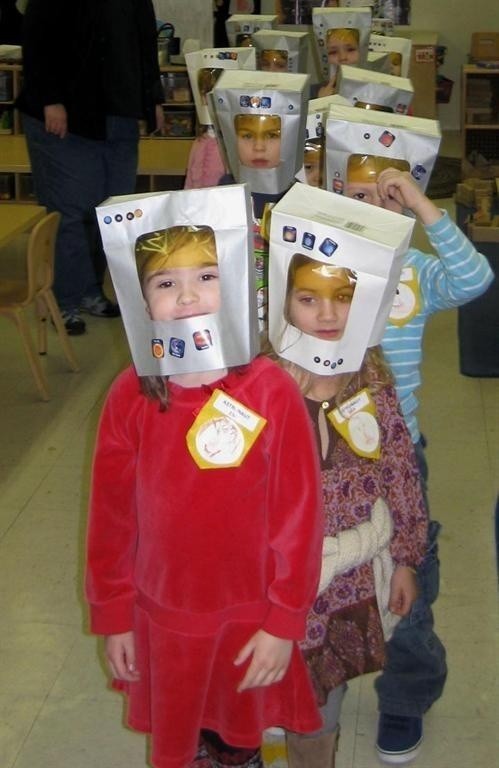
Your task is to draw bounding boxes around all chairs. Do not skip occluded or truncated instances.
[1,212,81,402]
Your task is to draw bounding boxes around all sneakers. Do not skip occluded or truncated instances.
[375,706,421,763]
[79,289,120,317]
[52,306,86,335]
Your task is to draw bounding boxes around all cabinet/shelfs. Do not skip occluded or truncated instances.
[0,64,198,199]
[460,64,499,243]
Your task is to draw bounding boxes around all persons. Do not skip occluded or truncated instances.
[84,226,324,767]
[256,264,430,767]
[346,153,496,765]
[219,113,303,334]
[183,28,401,189]
[14,0,166,335]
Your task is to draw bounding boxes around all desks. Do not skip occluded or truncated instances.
[0,203,46,249]
